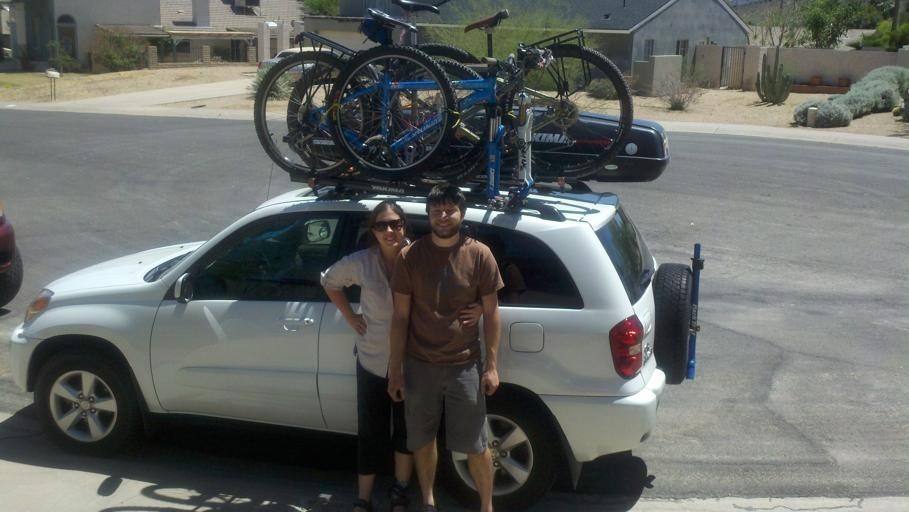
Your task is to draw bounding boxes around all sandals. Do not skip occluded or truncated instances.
[389,485,408,512]
[351,498,373,512]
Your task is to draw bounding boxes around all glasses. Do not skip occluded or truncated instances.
[371,219,404,232]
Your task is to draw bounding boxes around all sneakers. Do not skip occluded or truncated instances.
[420,496,437,512]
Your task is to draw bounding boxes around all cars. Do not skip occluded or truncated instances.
[312,76,670,180]
[10,181,704,511]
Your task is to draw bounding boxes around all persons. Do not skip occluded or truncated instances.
[385,183,499,512]
[320,200,483,512]
